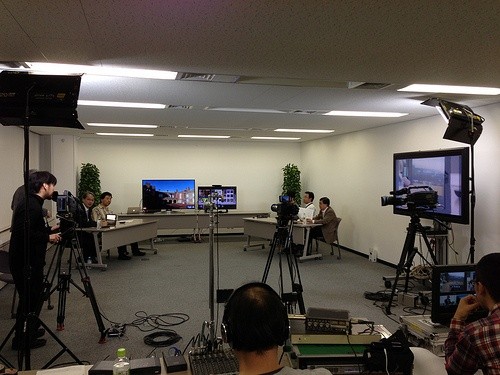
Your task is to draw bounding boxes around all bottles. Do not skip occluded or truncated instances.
[87,256,93,269]
[112,348,130,375]
[303,213,306,225]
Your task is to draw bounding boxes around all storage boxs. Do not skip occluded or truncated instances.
[400,316,451,357]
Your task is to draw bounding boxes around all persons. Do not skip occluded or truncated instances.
[9,169,50,232]
[445,253,500,375]
[278,191,316,256]
[220,282,332,375]
[295,197,338,259]
[8,171,60,350]
[91,192,146,260]
[73,191,107,269]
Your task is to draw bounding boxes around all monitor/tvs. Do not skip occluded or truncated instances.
[198,186,237,210]
[393,146,470,225]
[430,264,489,328]
[142,179,195,211]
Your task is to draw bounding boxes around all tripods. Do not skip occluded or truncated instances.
[262,226,306,315]
[0,93,108,372]
[386,208,438,315]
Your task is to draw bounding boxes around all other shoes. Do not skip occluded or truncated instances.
[77,254,99,263]
[118,255,132,261]
[11,335,46,351]
[133,251,146,257]
[34,328,46,338]
[277,248,288,255]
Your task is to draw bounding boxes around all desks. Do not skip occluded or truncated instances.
[243,217,322,262]
[118,211,270,242]
[71,219,158,271]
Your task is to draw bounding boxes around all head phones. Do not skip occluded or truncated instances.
[220,282,291,350]
